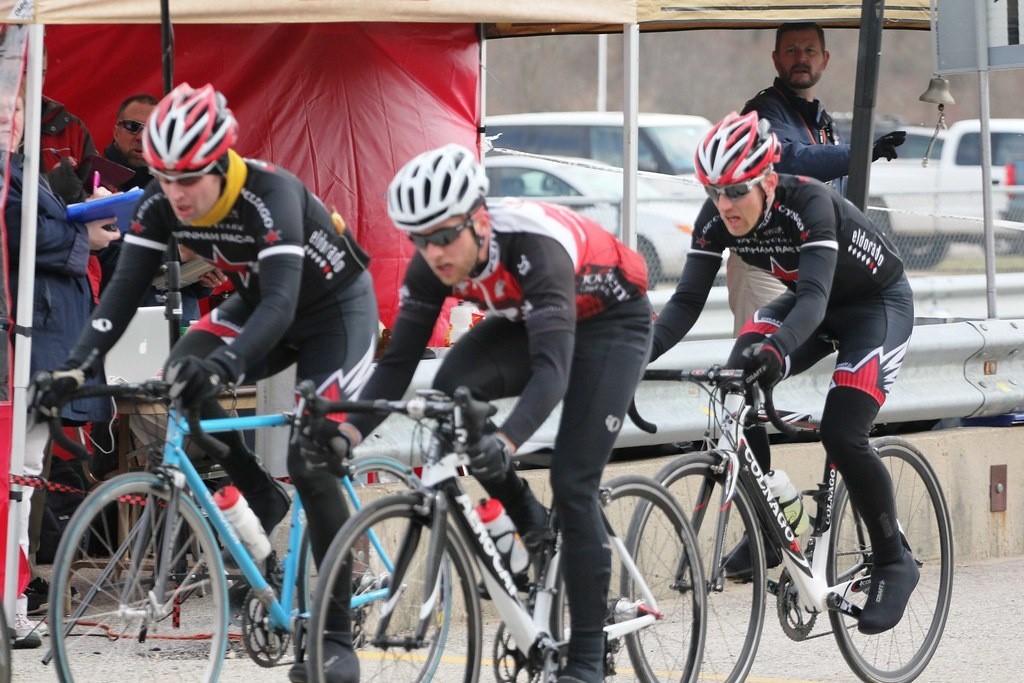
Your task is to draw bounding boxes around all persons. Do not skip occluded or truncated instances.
[297,142,654,683]
[0,70,161,649]
[726,20,907,340]
[647,109,920,634]
[38,79,378,683]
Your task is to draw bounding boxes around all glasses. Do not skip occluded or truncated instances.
[116,119,146,134]
[702,167,770,202]
[148,160,217,186]
[405,204,485,250]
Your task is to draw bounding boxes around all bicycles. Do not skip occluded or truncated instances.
[282,381,707,682]
[29,367,456,681]
[622,363,953,683]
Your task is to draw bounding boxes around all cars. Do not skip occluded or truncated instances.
[486,153,730,289]
[831,117,1023,268]
[484,110,724,187]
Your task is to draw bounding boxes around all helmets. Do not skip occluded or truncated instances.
[694,110,782,186]
[142,83,238,171]
[386,144,490,230]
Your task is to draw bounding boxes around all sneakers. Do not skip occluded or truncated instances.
[23,576,81,615]
[14,614,42,647]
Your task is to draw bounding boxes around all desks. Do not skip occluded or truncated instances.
[63,384,259,619]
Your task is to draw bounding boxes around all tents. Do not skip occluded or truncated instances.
[2,0,639,649]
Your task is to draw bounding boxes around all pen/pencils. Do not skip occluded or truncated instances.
[93,171,100,192]
[51,149,62,160]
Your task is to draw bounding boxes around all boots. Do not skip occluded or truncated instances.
[556,630,608,683]
[720,528,782,579]
[222,454,292,568]
[289,615,360,683]
[478,477,549,599]
[858,527,921,635]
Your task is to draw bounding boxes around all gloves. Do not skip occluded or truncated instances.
[742,337,785,393]
[25,359,88,424]
[871,131,906,163]
[460,434,509,489]
[165,357,231,412]
[300,419,353,473]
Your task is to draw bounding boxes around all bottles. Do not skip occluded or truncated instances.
[476,499,531,576]
[216,486,272,561]
[765,469,810,535]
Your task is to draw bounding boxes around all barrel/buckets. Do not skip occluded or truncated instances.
[103,306,171,384]
